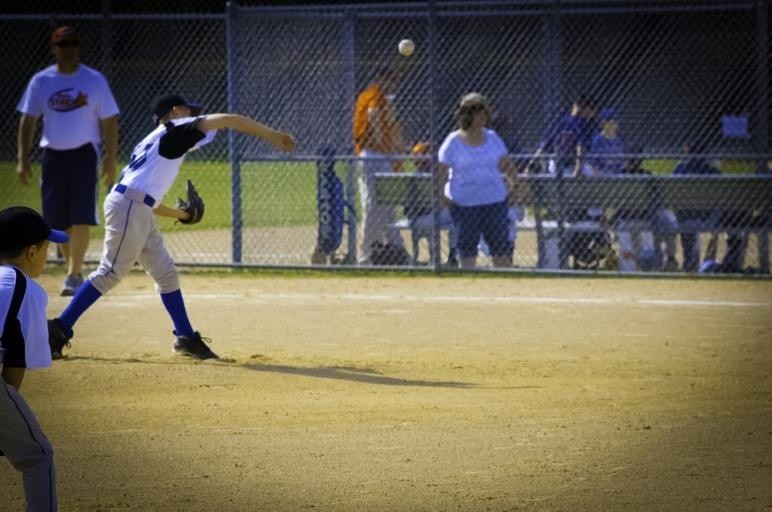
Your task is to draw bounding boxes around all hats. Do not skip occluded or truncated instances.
[0,205,71,262]
[50,26,78,44]
[458,91,489,111]
[148,93,205,124]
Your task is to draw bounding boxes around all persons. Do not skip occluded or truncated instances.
[1,206,69,512]
[14,23,120,295]
[47,93,296,359]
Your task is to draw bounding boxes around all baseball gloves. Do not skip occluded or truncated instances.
[178,180,204,223]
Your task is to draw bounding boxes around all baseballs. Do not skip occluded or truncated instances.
[398,40,415,57]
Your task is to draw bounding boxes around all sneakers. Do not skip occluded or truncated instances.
[170,328,220,366]
[59,272,85,297]
[40,313,75,360]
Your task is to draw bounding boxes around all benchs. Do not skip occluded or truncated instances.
[368,169,770,281]
